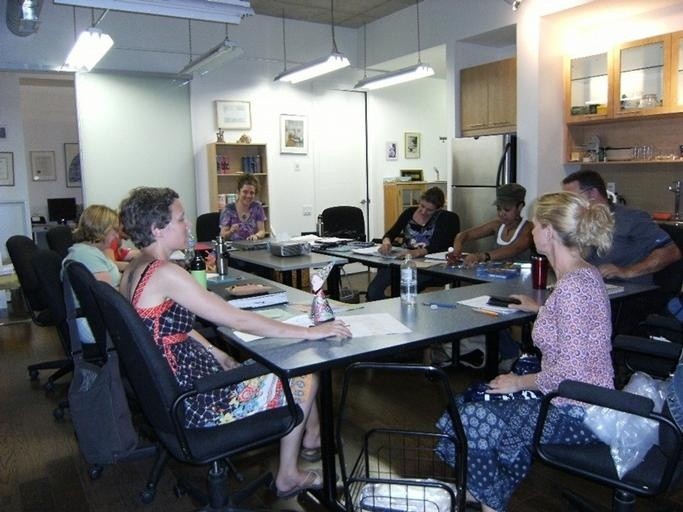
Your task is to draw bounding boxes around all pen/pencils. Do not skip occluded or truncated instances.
[472,308,498,316]
[422,303,456,308]
[309,324,350,328]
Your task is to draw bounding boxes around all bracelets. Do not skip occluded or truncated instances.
[484,252,491,262]
[255,234,260,241]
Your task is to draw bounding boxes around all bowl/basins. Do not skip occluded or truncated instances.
[621,97,640,109]
[572,102,607,116]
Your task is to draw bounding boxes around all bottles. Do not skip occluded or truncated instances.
[254,154,261,173]
[241,154,248,172]
[598,147,606,162]
[187,255,206,291]
[215,238,229,275]
[586,149,597,161]
[184,224,197,265]
[400,302,416,338]
[248,155,256,173]
[215,155,230,175]
[400,254,419,307]
[316,214,325,237]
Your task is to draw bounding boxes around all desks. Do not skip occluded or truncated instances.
[145,229,665,476]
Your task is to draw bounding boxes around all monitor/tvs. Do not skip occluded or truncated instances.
[47,197,76,225]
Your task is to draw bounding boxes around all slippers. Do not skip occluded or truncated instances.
[277,470,339,496]
[301,439,343,462]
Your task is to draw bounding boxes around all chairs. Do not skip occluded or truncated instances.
[88,283,308,512]
[531,332,682,512]
[195,211,225,242]
[318,207,368,297]
[67,262,172,502]
[45,225,75,256]
[6,235,94,427]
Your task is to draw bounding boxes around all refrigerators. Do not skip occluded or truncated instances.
[450,135,516,253]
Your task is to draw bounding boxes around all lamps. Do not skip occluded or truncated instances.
[60,0,118,75]
[269,2,353,88]
[172,2,242,88]
[350,2,436,93]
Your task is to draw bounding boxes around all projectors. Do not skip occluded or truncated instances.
[271,240,312,258]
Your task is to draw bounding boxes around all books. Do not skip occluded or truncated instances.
[207,278,288,310]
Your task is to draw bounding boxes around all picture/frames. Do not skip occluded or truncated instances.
[277,114,309,157]
[384,141,398,162]
[402,130,421,160]
[400,168,424,182]
[0,150,16,187]
[213,99,252,132]
[28,149,57,183]
[62,141,80,189]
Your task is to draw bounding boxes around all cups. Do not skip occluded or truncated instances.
[530,253,548,290]
[218,193,225,208]
[632,144,678,160]
[226,194,238,204]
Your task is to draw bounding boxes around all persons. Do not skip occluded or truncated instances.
[119,187,353,500]
[218,174,273,242]
[444,183,535,375]
[59,206,123,367]
[432,191,616,512]
[561,170,683,331]
[104,237,142,271]
[366,186,448,302]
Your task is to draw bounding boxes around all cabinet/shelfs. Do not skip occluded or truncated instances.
[563,114,683,166]
[458,56,516,137]
[205,142,271,236]
[563,31,671,123]
[381,180,448,238]
[669,32,683,112]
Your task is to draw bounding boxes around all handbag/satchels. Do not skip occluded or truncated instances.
[68,350,139,464]
[464,354,544,402]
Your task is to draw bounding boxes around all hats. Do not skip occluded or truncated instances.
[493,183,526,206]
